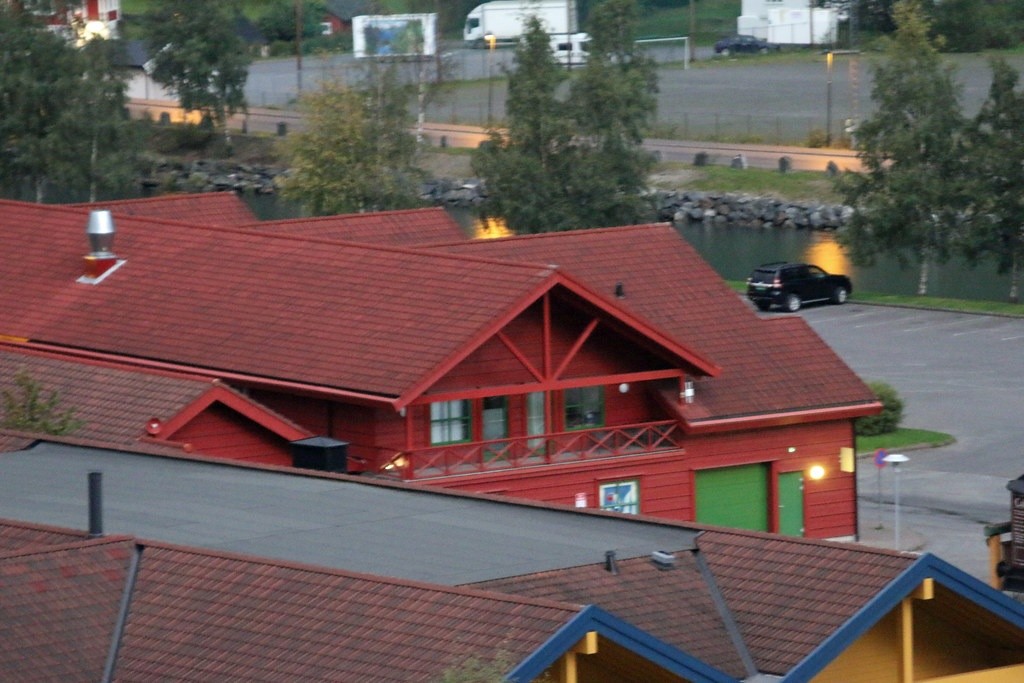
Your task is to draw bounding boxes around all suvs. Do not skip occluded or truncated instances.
[746,261,853,313]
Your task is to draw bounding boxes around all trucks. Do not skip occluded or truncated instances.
[463,0,579,48]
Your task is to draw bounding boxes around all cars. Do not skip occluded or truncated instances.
[712,33,781,57]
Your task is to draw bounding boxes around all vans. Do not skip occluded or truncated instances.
[512,32,593,67]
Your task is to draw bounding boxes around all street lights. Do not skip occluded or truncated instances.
[881,453,908,549]
